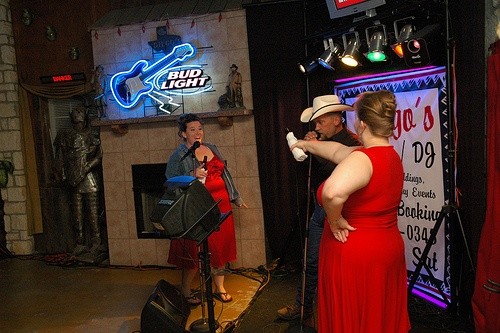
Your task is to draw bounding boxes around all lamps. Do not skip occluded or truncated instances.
[298,17,417,75]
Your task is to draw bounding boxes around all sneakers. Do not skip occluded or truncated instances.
[277,302,314,321]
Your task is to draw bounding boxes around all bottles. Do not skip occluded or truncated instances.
[284,127,307,161]
[198,156,208,186]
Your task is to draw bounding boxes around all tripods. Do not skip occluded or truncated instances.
[408,205,479,317]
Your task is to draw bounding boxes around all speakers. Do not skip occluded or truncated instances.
[141,281,191,333]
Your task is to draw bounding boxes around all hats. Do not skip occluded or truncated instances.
[300,94,354,123]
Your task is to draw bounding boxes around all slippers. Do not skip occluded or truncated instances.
[215,292,233,302]
[184,295,201,306]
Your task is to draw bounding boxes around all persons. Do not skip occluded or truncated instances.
[165,112,249,306]
[53,107,104,254]
[290,89,411,333]
[276,95,363,332]
[226,64,244,109]
[91,65,108,118]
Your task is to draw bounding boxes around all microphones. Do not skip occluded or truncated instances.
[181,141,200,161]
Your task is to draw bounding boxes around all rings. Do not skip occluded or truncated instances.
[337,232,341,234]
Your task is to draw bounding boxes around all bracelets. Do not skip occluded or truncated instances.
[328,215,343,224]
